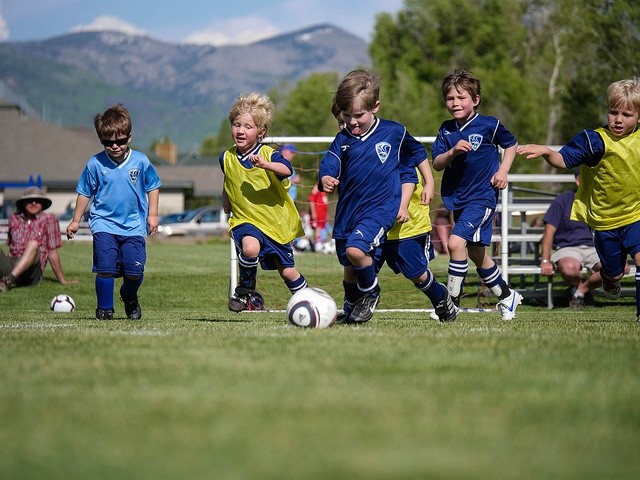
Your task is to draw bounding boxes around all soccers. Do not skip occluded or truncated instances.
[286,287,338,328]
[51,294,76,311]
[321,242,336,255]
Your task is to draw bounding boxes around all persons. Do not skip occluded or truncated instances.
[0,186,80,292]
[517,79,640,323]
[331,94,457,324]
[276,145,301,204]
[218,93,307,313]
[431,72,523,321]
[67,103,161,319]
[307,170,328,250]
[540,169,630,311]
[317,70,434,324]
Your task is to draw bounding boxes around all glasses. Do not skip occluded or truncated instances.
[98,133,133,147]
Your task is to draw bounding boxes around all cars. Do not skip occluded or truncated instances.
[156,205,230,241]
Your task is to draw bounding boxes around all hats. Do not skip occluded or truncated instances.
[16,186,52,213]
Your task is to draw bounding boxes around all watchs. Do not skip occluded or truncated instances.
[541,258,550,264]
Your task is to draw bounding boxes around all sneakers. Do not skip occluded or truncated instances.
[567,287,584,310]
[228,285,256,312]
[0,275,13,292]
[94,306,114,321]
[494,288,523,323]
[121,292,141,320]
[433,283,457,324]
[603,277,621,300]
[430,299,460,320]
[351,286,381,324]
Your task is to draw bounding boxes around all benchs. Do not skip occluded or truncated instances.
[492,175,636,308]
